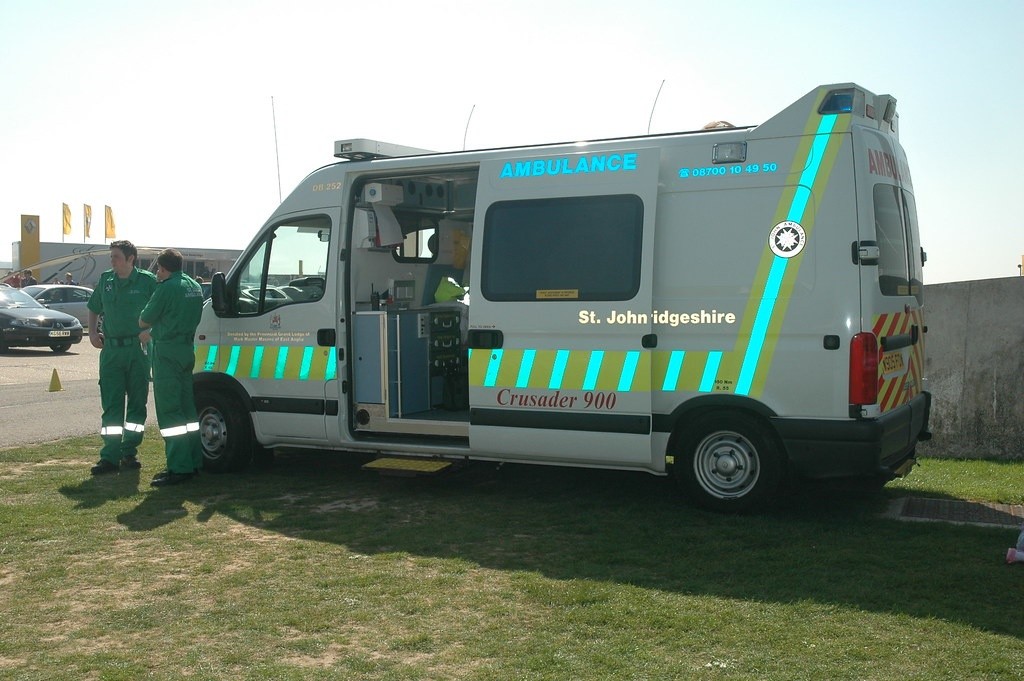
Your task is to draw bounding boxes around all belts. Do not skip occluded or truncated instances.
[103,336,139,346]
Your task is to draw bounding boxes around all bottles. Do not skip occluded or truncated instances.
[378,299,389,312]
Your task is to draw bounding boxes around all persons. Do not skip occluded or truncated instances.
[53,272,79,285]
[4,269,37,288]
[87,240,161,473]
[138,248,203,486]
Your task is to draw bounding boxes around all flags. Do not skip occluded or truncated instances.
[105,206,116,239]
[84,204,91,238]
[63,203,71,235]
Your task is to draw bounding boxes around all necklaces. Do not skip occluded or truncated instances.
[25,277,31,285]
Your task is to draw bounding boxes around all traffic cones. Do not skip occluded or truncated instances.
[46,367,64,392]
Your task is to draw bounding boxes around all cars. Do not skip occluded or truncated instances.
[0,283,84,354]
[13,284,104,333]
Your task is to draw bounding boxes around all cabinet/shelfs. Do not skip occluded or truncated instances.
[349,306,462,419]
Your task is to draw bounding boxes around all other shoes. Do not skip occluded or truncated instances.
[121,456,142,468]
[150,472,193,486]
[90,459,119,475]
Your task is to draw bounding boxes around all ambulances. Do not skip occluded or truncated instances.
[189,80,934,516]
